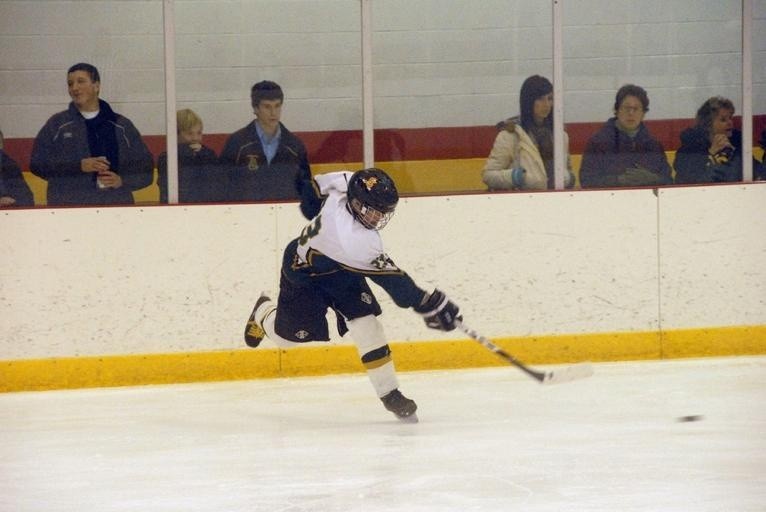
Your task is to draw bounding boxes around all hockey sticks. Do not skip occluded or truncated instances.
[454,318,593,383]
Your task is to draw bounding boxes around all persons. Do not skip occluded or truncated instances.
[157,108,225,205]
[480,75,580,190]
[0,129,34,208]
[217,81,314,201]
[759,125,766,179]
[30,63,155,208]
[673,91,763,182]
[577,83,675,189]
[241,166,464,418]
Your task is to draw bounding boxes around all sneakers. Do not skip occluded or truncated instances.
[244,296,273,348]
[381,389,416,417]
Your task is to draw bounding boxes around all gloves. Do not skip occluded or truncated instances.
[414,288,463,330]
[617,162,659,187]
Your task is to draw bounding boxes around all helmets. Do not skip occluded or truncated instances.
[347,167,398,231]
